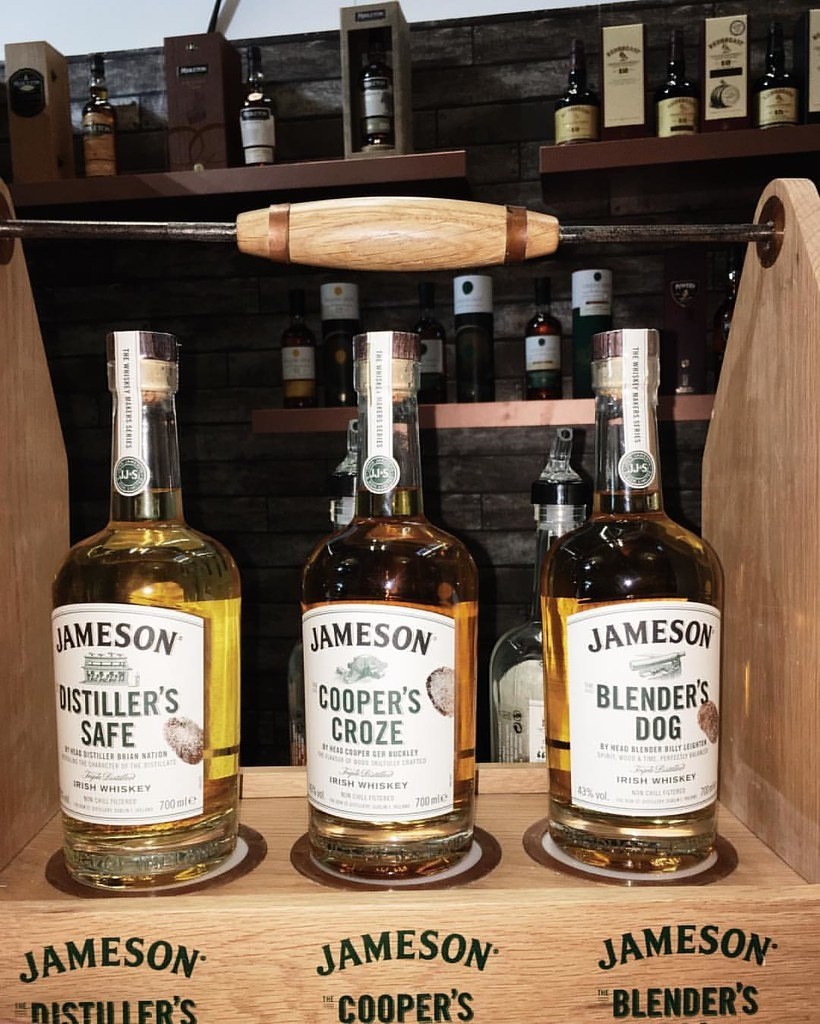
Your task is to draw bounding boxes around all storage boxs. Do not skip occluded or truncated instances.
[4,41,73,184]
[164,32,240,172]
[342,1,413,160]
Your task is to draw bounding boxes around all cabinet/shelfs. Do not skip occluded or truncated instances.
[0,112,820,426]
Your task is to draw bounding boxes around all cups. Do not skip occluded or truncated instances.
[453,274,497,403]
[571,269,614,400]
[319,282,361,408]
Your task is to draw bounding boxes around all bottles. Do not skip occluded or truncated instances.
[523,276,564,400]
[280,289,317,408]
[298,331,481,881]
[552,38,602,146]
[412,281,448,404]
[487,427,587,763]
[654,31,699,138]
[755,17,800,130]
[50,331,243,894]
[286,419,359,764]
[712,245,741,393]
[82,55,118,178]
[538,325,725,875]
[358,47,393,145]
[238,47,276,167]
